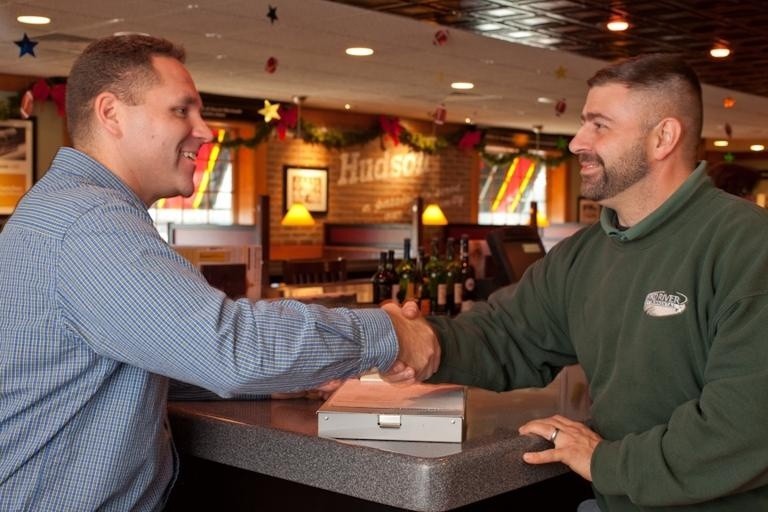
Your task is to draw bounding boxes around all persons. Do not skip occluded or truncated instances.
[385,57,768,512]
[0,34,443,512]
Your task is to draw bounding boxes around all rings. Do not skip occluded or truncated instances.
[551,428,560,442]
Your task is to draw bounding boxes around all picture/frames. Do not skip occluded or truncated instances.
[0,116,38,220]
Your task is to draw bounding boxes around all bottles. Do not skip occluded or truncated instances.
[373,234,477,317]
